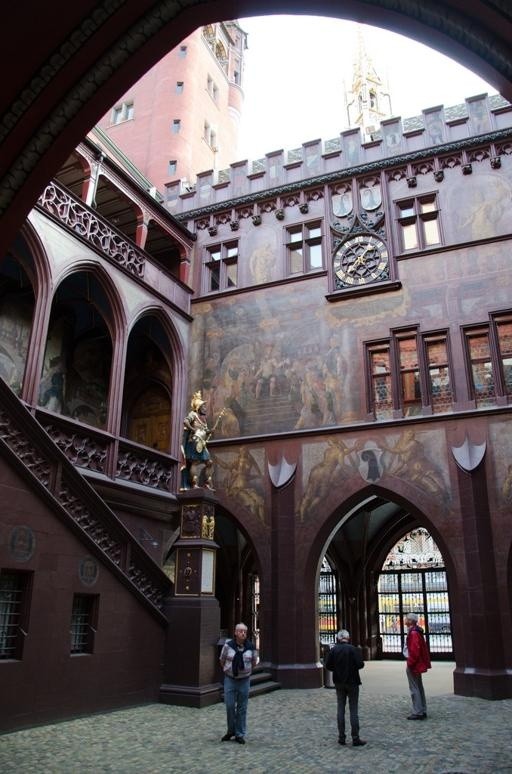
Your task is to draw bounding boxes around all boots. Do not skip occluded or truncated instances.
[338,735,346,745]
[353,736,366,746]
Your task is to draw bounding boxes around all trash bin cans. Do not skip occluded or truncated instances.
[324,644,337,688]
[216,638,232,683]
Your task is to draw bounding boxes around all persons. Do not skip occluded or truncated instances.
[404,613,432,721]
[220,622,260,744]
[182,392,217,493]
[325,628,368,746]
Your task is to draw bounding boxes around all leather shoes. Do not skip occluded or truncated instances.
[236,737,245,744]
[222,732,234,741]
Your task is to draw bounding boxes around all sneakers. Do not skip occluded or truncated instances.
[407,712,427,720]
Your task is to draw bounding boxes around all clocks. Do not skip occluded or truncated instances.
[330,231,388,286]
